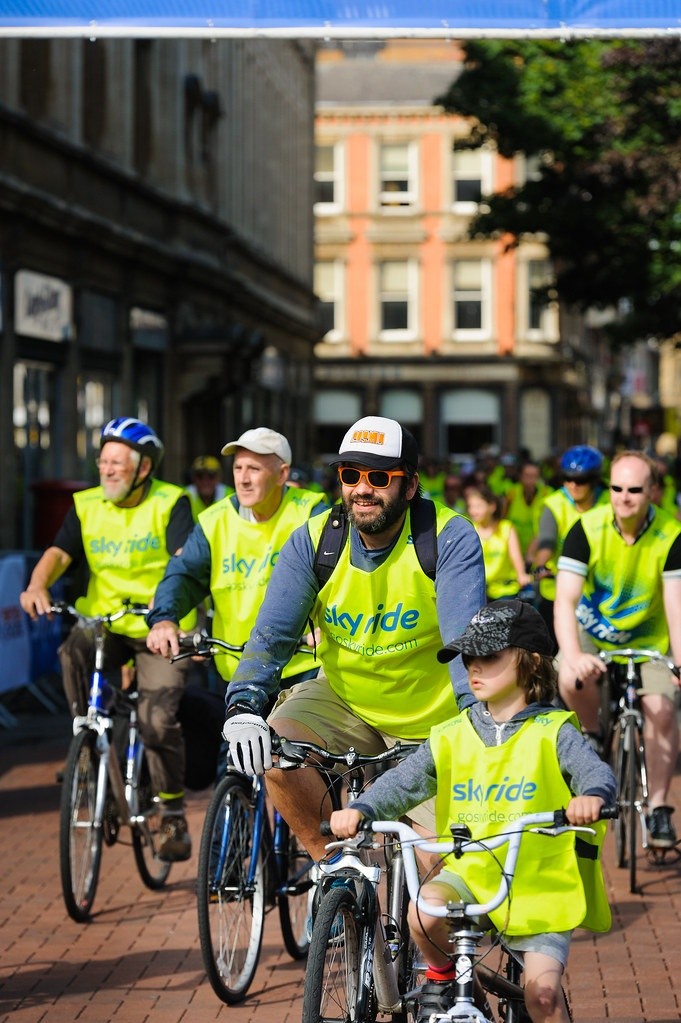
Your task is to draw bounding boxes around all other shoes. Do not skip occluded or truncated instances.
[304,855,347,948]
[583,723,605,760]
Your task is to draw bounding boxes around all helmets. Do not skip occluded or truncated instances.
[192,455,220,472]
[561,445,603,475]
[99,417,165,481]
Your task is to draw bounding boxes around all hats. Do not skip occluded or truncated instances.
[435,599,548,664]
[328,416,419,475]
[220,427,292,466]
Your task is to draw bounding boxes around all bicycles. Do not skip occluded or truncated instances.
[577,647,681,892]
[221,735,429,1023]
[30,597,173,921]
[166,632,333,1004]
[322,803,620,1023]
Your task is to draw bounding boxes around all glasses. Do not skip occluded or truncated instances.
[95,457,127,472]
[564,475,588,486]
[337,464,405,489]
[609,485,644,495]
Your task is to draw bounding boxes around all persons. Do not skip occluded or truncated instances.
[19,416,681,848]
[329,598,617,1023]
[222,416,487,971]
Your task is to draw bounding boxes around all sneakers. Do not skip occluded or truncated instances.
[53,745,92,782]
[645,804,677,848]
[415,978,459,1023]
[158,810,192,862]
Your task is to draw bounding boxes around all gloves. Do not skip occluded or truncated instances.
[223,701,273,776]
